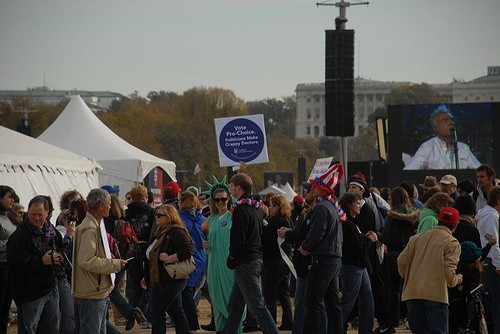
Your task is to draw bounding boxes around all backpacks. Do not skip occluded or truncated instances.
[127,207,151,244]
[106,216,140,261]
[105,232,121,260]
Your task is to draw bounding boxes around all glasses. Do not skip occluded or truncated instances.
[213,197,229,203]
[152,213,166,218]
[17,211,22,214]
[124,197,132,202]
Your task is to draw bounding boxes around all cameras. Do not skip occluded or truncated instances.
[66,213,80,223]
[484,233,493,239]
[41,246,63,271]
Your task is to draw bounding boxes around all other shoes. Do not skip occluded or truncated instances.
[374,326,397,334]
[242,320,261,333]
[200,324,216,331]
[125,307,176,331]
[277,324,293,331]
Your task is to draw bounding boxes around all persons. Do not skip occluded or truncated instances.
[0,164,500,334]
[402,105,482,168]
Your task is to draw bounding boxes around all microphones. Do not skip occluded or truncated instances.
[450,127,458,145]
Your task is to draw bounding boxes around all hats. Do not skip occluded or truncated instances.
[459,241,482,262]
[438,207,460,224]
[349,172,368,190]
[310,162,343,194]
[186,186,198,194]
[418,176,440,192]
[100,185,120,193]
[438,175,457,186]
[164,181,182,193]
[292,195,306,202]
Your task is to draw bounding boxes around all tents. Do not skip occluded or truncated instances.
[36,95,177,210]
[0,126,104,227]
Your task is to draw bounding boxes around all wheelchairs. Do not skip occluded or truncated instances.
[452,283,500,334]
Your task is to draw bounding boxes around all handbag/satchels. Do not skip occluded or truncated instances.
[162,228,196,280]
